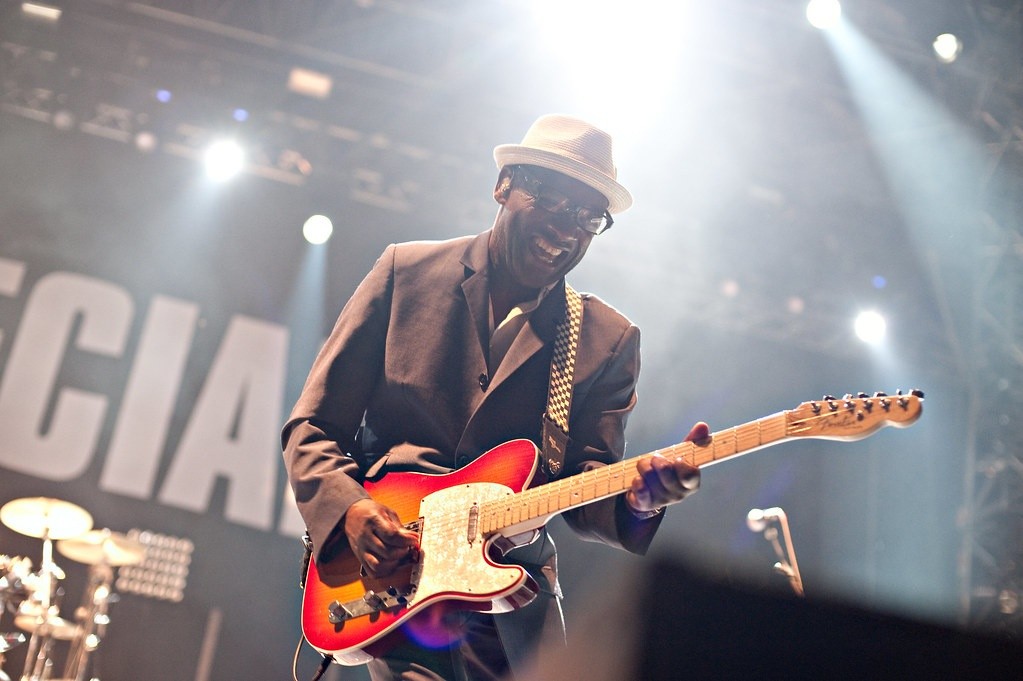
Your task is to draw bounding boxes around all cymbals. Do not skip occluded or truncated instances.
[0,497,93,541]
[57,528,148,568]
[13,612,80,640]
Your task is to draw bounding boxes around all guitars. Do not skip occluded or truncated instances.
[300,386,928,668]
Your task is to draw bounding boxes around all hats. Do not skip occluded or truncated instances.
[494,114,632,215]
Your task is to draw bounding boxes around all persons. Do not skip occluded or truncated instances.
[281,112,709,681]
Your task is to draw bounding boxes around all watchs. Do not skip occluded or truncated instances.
[625,491,665,520]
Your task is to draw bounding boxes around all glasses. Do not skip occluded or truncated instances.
[517,165,615,235]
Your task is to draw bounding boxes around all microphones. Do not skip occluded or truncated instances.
[746,507,785,532]
